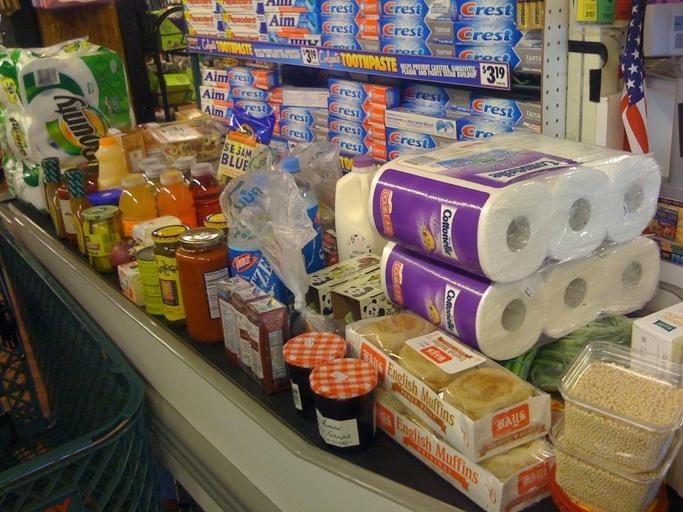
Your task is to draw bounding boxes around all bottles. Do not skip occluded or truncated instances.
[64,168,93,255]
[41,157,62,237]
[97,135,222,236]
[280,156,320,226]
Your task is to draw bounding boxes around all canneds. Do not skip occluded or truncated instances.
[281,333,378,453]
[55,181,96,241]
[79,188,125,275]
[135,208,231,346]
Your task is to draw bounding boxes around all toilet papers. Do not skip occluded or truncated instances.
[367,131,663,361]
[0,40,138,211]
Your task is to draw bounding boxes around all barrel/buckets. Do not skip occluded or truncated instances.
[333,156,395,261]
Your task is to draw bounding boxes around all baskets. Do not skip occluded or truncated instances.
[0,227,163,512]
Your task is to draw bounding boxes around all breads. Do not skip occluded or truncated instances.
[355,312,557,483]
